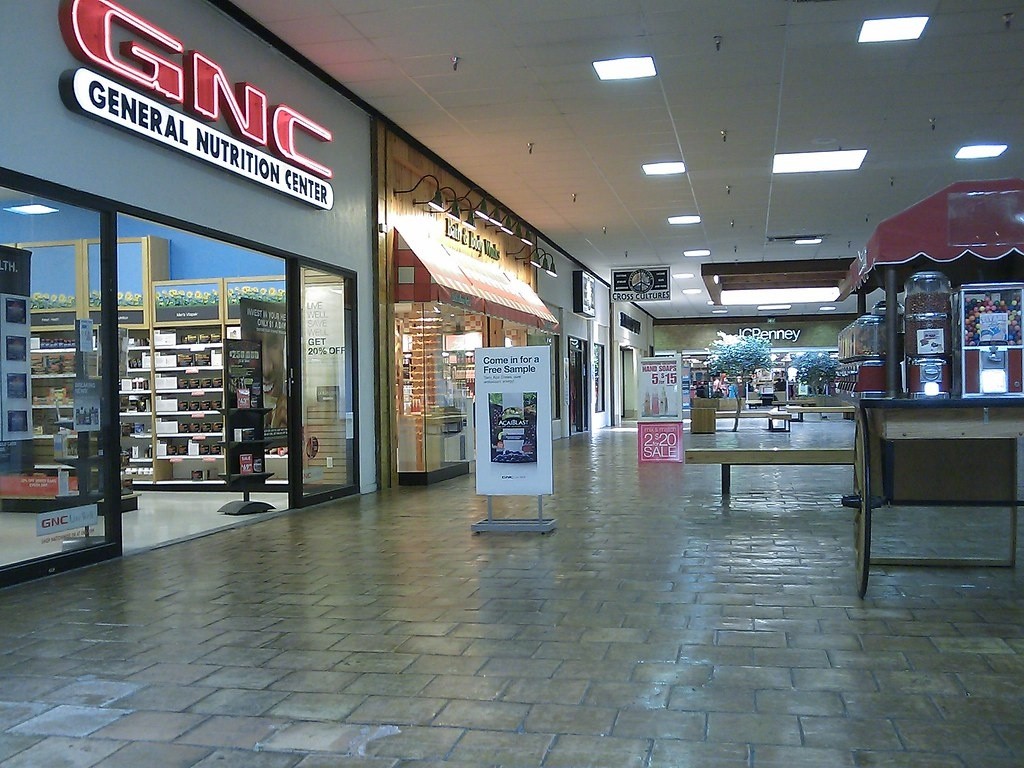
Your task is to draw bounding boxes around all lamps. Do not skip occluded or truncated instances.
[392,174,558,278]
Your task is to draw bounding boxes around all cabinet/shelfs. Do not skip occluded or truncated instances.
[217,405,275,485]
[408,317,444,406]
[224,276,286,325]
[30,339,223,473]
[151,273,223,325]
[15,235,169,333]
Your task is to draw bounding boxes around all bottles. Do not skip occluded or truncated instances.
[148,444,152,458]
[455,380,472,399]
[80,406,99,424]
[41,338,75,349]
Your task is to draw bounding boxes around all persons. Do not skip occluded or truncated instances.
[713,377,721,393]
[241,329,287,428]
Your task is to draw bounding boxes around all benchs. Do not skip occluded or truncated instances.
[787,406,856,420]
[684,446,857,496]
[772,401,816,411]
[715,412,792,432]
